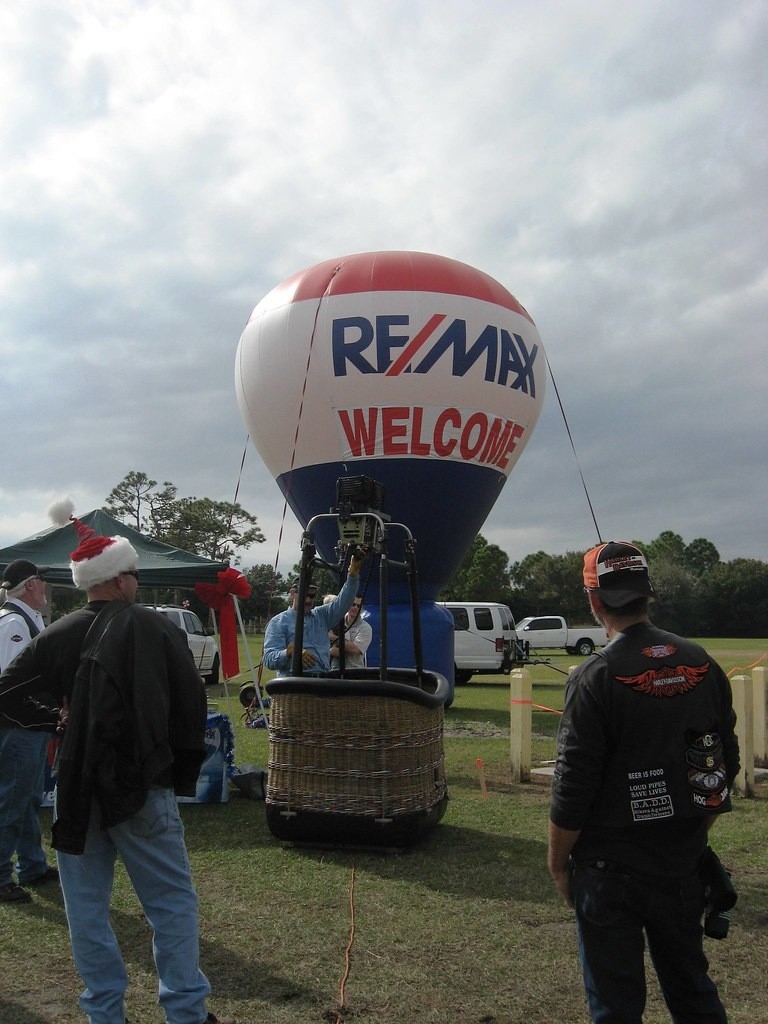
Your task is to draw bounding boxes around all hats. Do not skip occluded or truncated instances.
[292,576,321,589]
[583,540,657,605]
[3,558,49,590]
[47,497,138,588]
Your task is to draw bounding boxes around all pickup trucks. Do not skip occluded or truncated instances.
[514,616,610,657]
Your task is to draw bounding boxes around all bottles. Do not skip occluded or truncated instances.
[703,868,731,938]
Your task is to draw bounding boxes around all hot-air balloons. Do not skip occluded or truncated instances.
[231,252,547,856]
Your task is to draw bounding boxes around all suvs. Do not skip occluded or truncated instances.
[434,601,542,686]
[137,603,220,685]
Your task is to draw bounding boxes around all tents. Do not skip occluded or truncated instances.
[0,505,237,1024]
[0,509,229,609]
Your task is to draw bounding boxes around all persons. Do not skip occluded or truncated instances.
[550,541,738,1024]
[323,592,372,668]
[262,547,368,677]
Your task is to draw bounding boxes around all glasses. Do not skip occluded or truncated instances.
[118,570,139,580]
[295,592,316,599]
[31,575,46,582]
[353,603,360,608]
[584,586,597,594]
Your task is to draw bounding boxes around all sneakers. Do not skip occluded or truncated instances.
[17,867,59,885]
[0,882,33,903]
